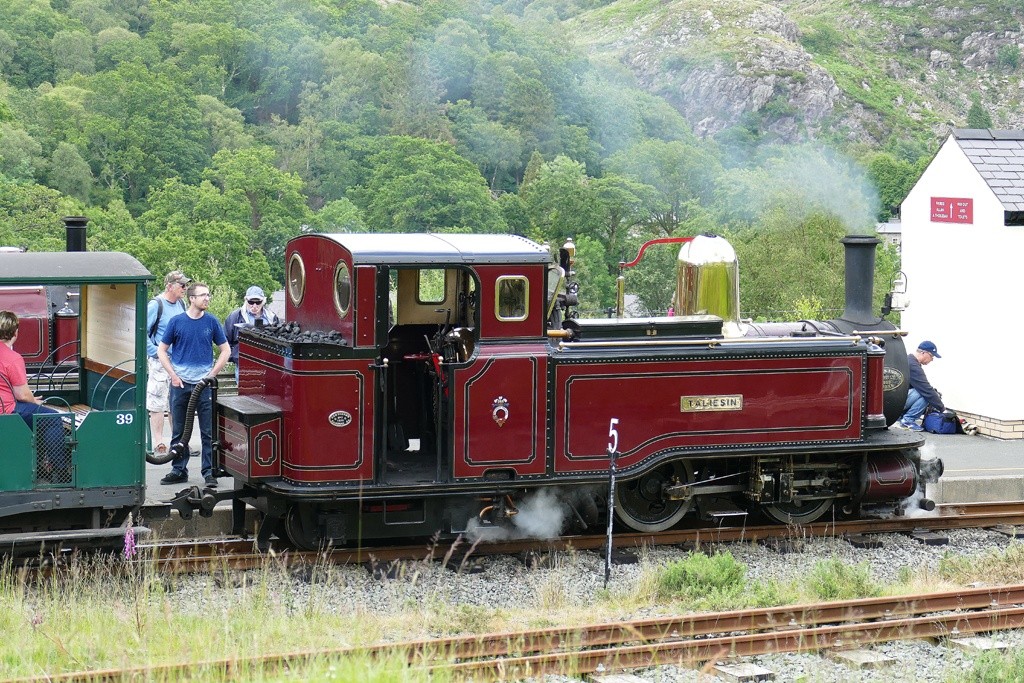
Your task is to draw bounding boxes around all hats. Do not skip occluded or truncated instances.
[246,286,264,298]
[164,271,192,284]
[918,341,942,358]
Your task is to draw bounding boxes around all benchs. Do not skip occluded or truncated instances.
[33,389,137,432]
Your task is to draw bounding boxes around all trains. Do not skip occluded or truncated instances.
[0,216,928,567]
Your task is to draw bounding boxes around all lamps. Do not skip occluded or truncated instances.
[890,269,911,312]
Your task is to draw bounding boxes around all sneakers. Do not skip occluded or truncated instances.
[896,419,924,432]
[204,473,218,487]
[153,442,167,457]
[189,446,200,457]
[159,472,188,485]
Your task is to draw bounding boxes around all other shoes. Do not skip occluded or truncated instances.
[37,465,71,484]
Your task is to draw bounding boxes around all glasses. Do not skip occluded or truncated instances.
[190,293,210,297]
[246,299,263,305]
[175,283,189,289]
[926,351,934,359]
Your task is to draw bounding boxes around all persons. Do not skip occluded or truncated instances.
[898,341,946,431]
[224,286,279,386]
[157,283,233,487]
[0,311,71,484]
[146,270,200,458]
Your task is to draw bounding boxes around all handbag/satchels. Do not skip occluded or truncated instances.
[922,408,966,434]
